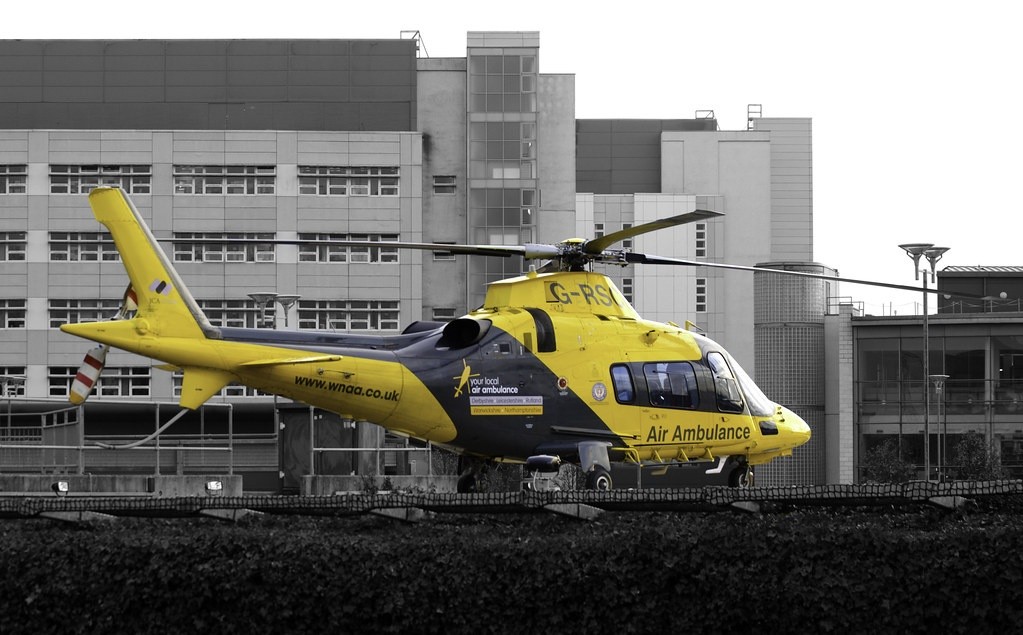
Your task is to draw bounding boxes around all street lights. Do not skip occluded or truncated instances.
[928,374,950,483]
[898,242,952,498]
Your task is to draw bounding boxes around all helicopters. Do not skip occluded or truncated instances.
[59,180,815,491]
